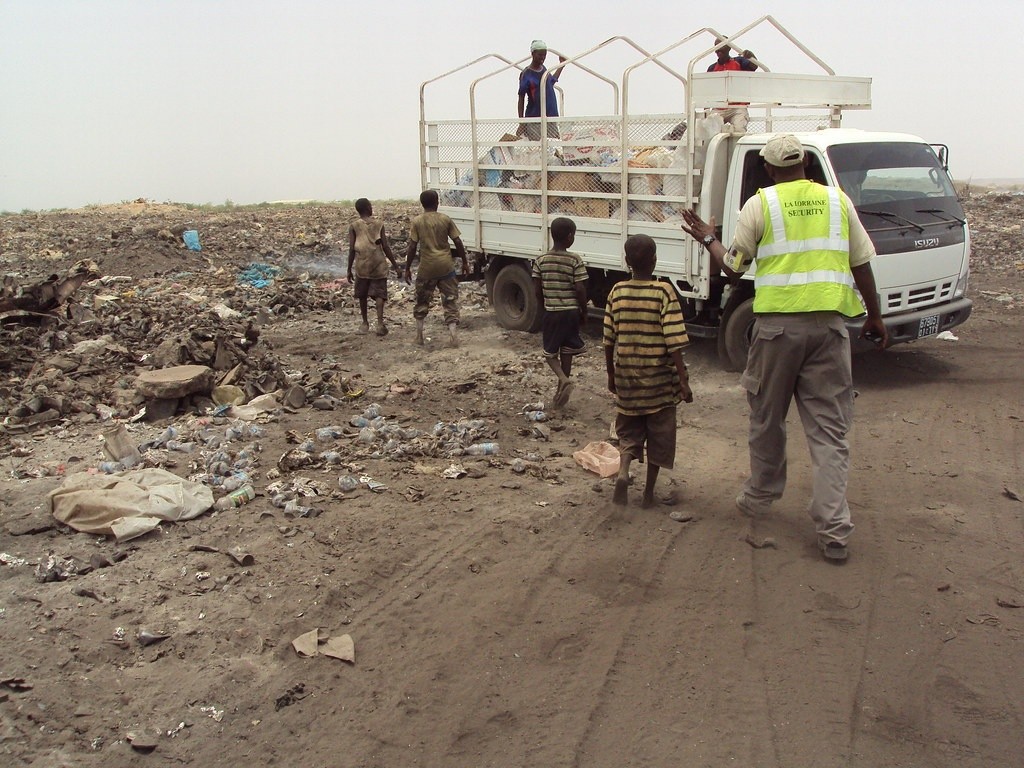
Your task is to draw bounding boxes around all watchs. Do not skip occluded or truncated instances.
[703,234,718,248]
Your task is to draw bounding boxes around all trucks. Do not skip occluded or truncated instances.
[420,13,976,374]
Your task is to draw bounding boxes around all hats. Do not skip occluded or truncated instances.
[530,40,547,53]
[759,133,805,166]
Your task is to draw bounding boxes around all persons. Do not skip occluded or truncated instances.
[679,132,887,558]
[517,41,566,141]
[602,234,693,508]
[404,190,470,347]
[704,35,758,133]
[532,217,591,405]
[346,198,401,336]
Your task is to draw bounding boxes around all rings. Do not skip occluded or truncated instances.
[691,223,694,226]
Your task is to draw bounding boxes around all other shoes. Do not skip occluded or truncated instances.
[356,321,369,335]
[413,336,423,344]
[825,543,849,560]
[736,495,771,520]
[451,332,459,348]
[553,381,574,406]
[377,324,388,336]
